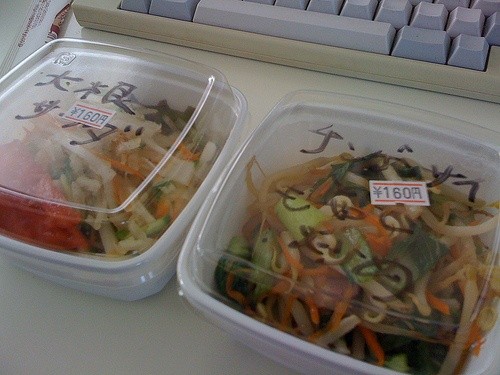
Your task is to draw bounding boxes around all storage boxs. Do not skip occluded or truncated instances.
[0,38,500,375]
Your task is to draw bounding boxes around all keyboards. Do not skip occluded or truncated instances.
[69,0,500,103]
